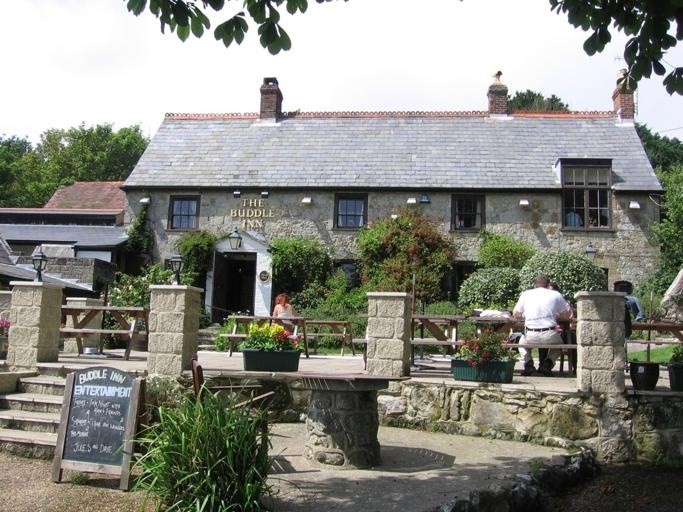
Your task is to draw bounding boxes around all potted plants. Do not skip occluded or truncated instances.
[665,346,683,390]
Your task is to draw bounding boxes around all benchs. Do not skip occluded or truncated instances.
[220,332,355,357]
[411,337,577,377]
[60,328,138,360]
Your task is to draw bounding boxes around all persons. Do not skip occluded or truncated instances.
[271,294,300,335]
[513,276,574,375]
[565,206,584,226]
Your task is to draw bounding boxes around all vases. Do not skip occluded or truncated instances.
[241,348,301,371]
[630,360,664,389]
[451,359,512,382]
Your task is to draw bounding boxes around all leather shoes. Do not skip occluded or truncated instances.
[521,365,553,376]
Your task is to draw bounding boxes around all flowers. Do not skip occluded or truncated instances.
[449,326,516,367]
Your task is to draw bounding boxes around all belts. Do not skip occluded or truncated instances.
[524,327,554,332]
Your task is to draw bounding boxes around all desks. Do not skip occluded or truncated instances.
[626,320,682,363]
[228,315,351,358]
[62,304,149,358]
[412,313,574,375]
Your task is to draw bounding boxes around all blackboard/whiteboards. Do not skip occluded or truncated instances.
[54,366,144,475]
